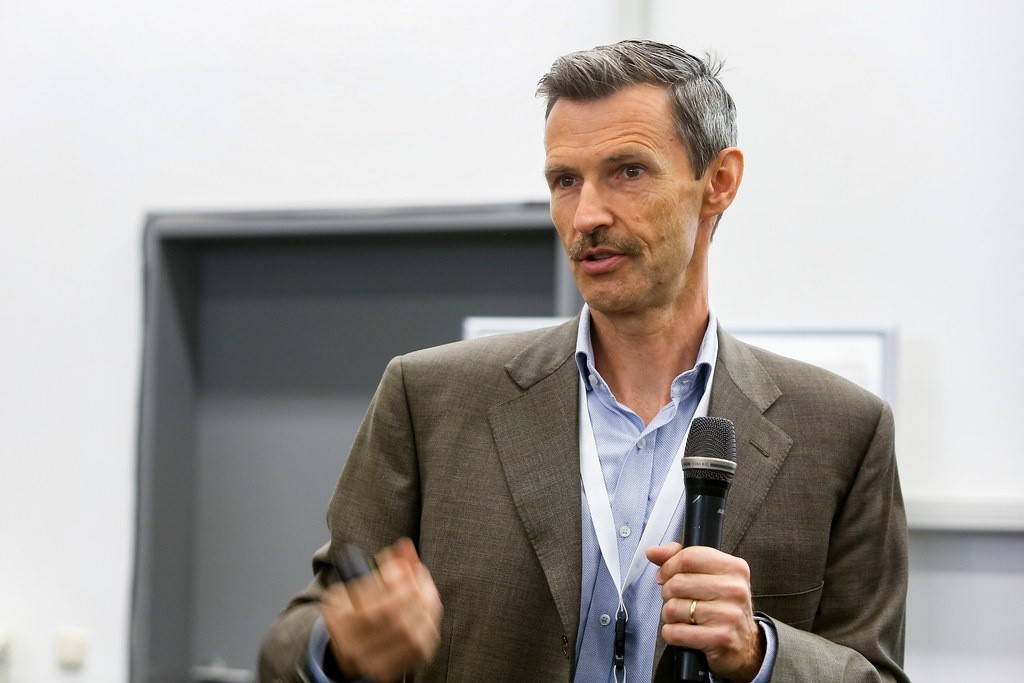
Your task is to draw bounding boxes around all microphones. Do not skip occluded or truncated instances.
[680,416,738,683]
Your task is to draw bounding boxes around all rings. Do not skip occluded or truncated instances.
[690,599,698,624]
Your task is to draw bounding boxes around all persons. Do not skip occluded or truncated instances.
[252,38,911,683]
